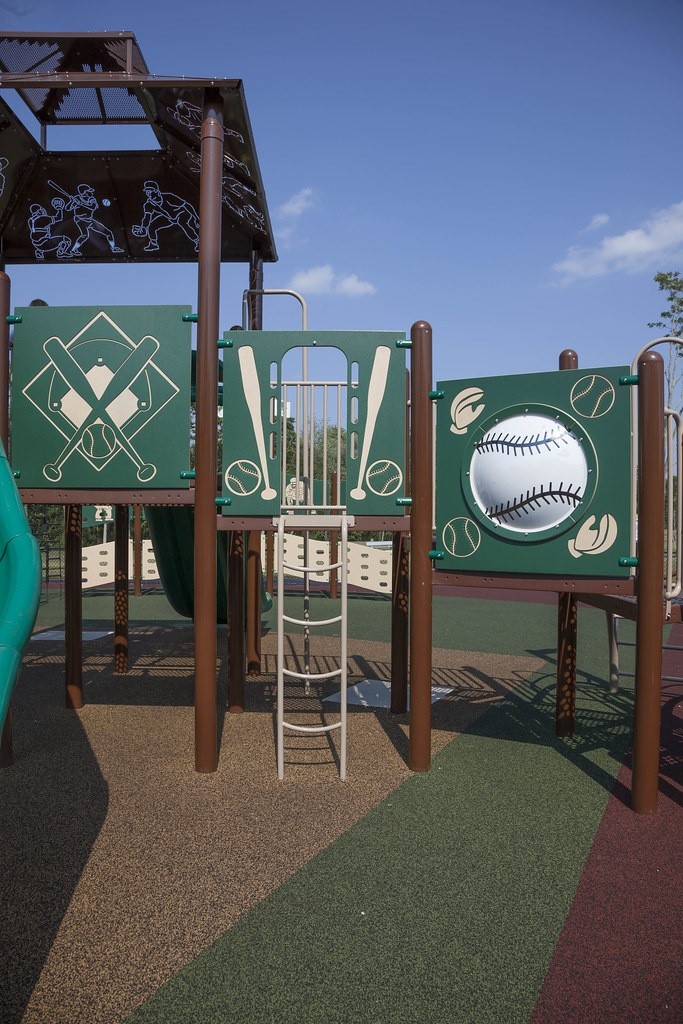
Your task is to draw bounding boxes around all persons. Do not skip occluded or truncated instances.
[9,298,48,348]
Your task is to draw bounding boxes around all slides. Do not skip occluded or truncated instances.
[146,504,273,617]
